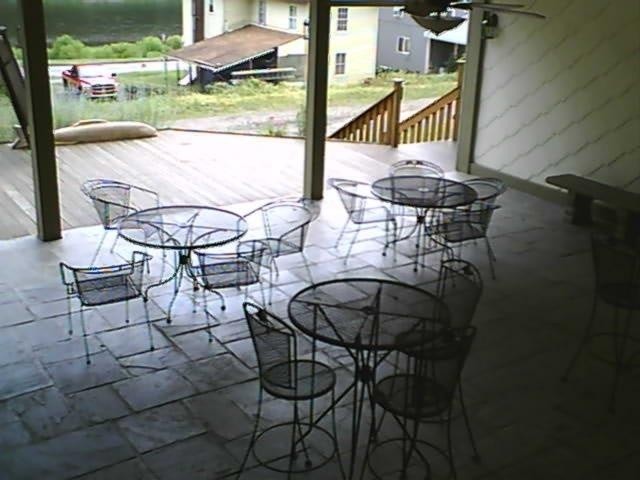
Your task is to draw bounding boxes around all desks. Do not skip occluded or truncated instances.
[546,174,639,242]
[370,174,478,273]
[117,205,248,324]
[288,278,451,479]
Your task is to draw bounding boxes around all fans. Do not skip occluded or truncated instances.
[411,10,466,36]
[326,0,547,24]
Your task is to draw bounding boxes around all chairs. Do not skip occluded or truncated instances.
[234,302,348,480]
[384,258,484,397]
[358,320,477,480]
[562,230,640,415]
[326,177,398,265]
[237,197,321,306]
[79,178,166,269]
[389,160,445,246]
[59,251,154,364]
[192,239,267,343]
[434,178,508,281]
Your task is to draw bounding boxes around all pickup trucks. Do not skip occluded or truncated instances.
[62,64,119,100]
